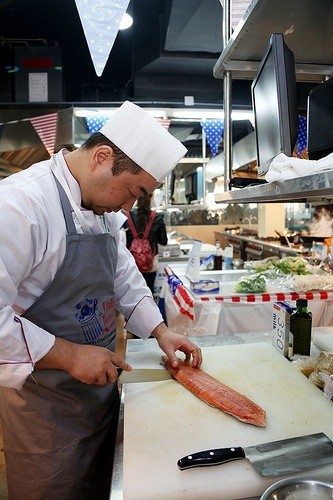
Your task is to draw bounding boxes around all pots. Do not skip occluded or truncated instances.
[279,234,300,245]
[301,235,324,248]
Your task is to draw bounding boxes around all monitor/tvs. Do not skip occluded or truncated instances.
[251,33,296,177]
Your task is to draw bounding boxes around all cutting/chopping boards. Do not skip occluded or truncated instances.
[122,343,333,500]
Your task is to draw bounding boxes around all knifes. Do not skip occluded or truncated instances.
[177,432,333,477]
[116,367,172,384]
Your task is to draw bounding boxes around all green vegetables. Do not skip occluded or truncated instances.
[233,258,314,294]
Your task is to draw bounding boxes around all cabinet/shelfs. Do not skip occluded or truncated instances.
[213,0,333,205]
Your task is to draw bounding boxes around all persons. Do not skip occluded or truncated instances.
[122,191,169,291]
[0,101,204,500]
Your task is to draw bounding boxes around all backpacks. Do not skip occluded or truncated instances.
[125,210,157,272]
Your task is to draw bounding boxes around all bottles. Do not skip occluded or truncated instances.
[213,241,222,271]
[287,299,312,360]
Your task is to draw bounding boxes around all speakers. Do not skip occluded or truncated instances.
[306,77,333,160]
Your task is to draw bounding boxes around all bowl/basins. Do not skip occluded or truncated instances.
[259,477,333,500]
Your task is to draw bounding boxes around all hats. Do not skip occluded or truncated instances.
[99,100,188,183]
